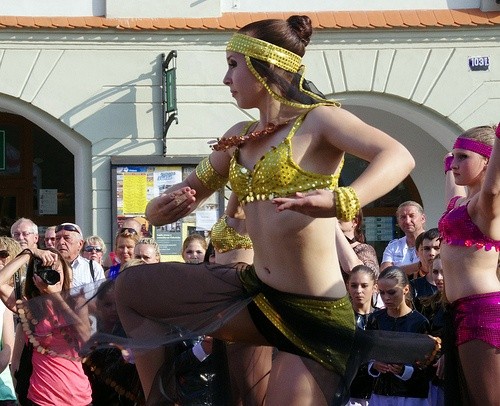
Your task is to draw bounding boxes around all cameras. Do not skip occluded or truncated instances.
[34,261,60,285]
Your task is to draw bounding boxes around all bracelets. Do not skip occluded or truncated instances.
[443,156,453,174]
[334,185,359,222]
[495,122,500,139]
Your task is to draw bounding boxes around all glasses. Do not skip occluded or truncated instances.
[82,246,102,252]
[55,225,80,233]
[0,250,10,259]
[12,231,34,237]
[121,228,137,235]
[44,237,56,242]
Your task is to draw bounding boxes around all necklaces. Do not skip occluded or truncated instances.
[207,115,300,151]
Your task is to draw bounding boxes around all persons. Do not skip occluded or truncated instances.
[335,201,444,406]
[16,15,441,406]
[439,121,500,406]
[210,190,273,406]
[0,218,215,406]
